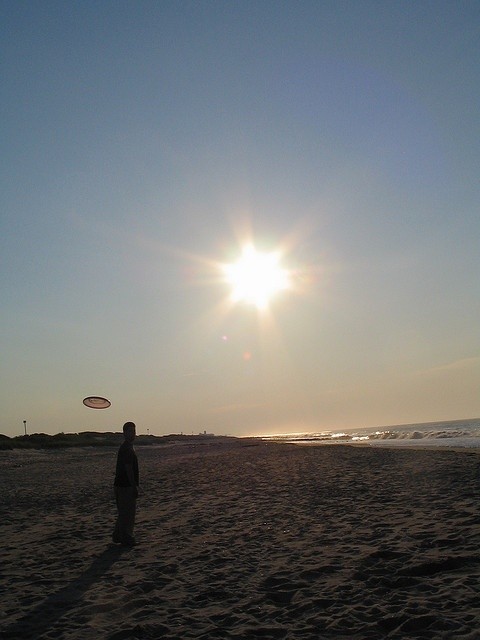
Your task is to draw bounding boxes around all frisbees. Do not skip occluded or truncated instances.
[83,397,111,409]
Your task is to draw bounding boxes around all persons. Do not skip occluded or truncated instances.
[112,422,139,548]
